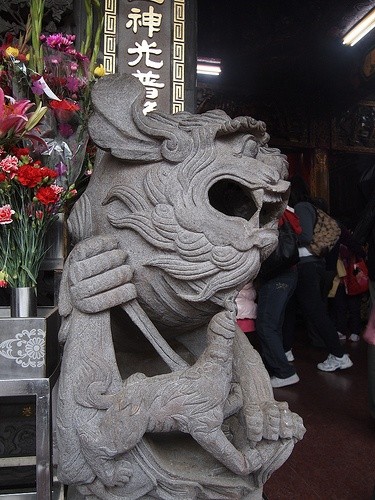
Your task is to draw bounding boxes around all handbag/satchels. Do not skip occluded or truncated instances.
[342,255,369,295]
[304,205,342,258]
[271,212,300,269]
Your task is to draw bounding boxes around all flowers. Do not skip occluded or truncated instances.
[0,0,106,287]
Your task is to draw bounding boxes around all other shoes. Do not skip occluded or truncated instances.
[349,334,360,342]
[337,331,347,340]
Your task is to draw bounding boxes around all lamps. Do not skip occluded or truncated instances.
[341,7,375,48]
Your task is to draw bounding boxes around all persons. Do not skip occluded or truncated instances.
[236,175,375,436]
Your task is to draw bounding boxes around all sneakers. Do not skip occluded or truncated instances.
[317,353,353,371]
[270,372,300,388]
[284,347,295,362]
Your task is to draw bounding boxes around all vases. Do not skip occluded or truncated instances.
[11,288,37,318]
